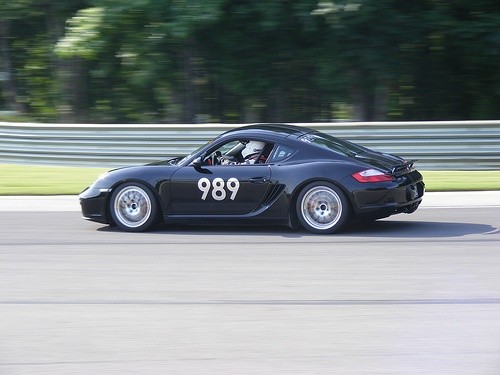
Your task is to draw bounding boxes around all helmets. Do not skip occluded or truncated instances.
[241,141,265,159]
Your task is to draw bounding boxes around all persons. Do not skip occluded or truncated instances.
[217,140,266,165]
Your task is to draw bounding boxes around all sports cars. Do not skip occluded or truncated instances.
[78,124,426,235]
